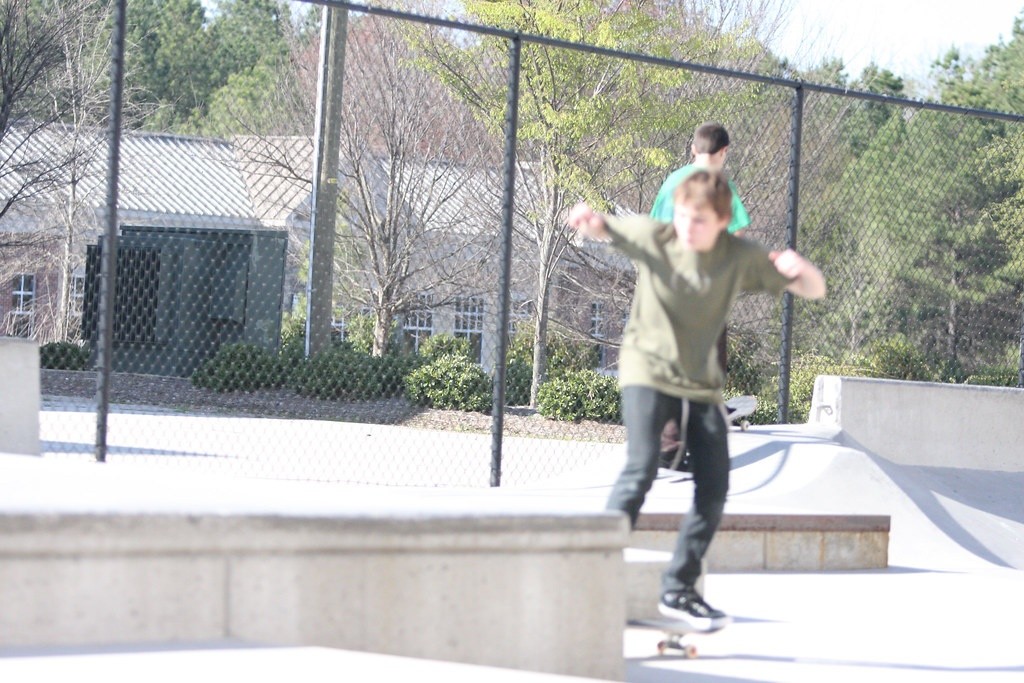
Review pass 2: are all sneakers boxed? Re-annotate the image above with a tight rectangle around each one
[656,590,728,632]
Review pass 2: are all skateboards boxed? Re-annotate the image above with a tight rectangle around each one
[660,392,758,460]
[623,613,723,659]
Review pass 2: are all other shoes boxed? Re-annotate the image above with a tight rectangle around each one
[656,447,691,472]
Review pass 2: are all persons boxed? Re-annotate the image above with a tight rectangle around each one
[567,172,827,630]
[647,120,748,236]
